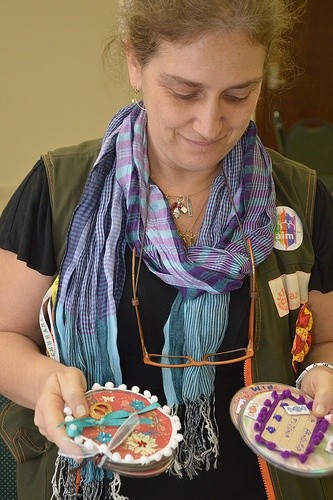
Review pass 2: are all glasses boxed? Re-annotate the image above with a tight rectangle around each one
[132,238,256,368]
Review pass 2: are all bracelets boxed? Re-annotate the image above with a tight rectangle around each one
[295,362,333,389]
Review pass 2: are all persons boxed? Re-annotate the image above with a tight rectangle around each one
[1,0,333,500]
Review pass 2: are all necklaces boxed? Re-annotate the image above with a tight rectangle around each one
[164,184,211,247]
